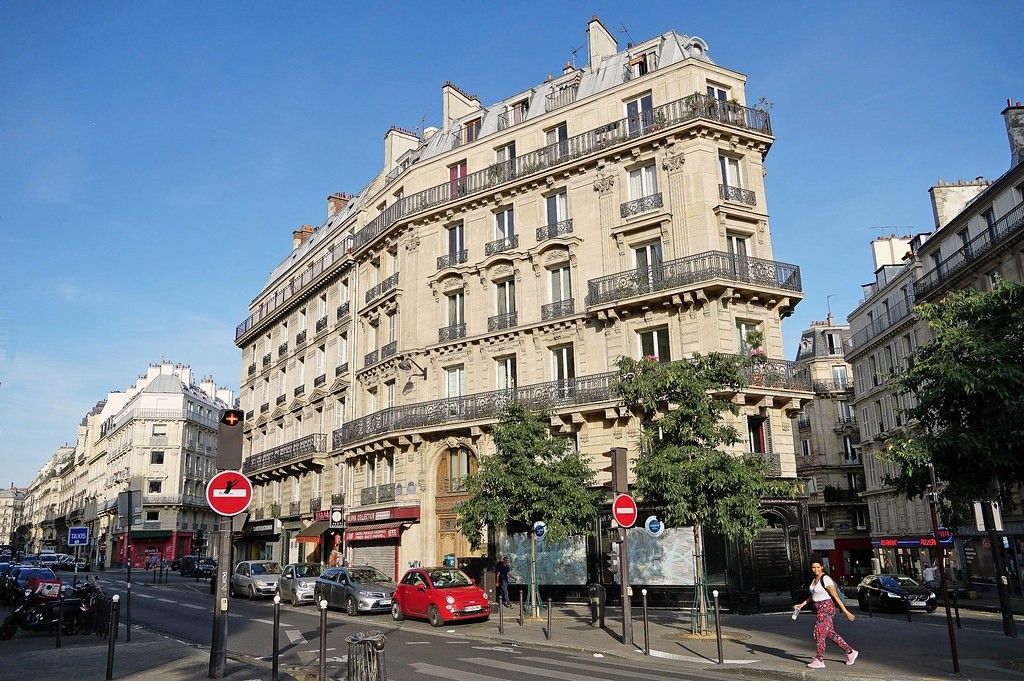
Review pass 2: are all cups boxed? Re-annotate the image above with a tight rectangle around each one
[792,608,800,619]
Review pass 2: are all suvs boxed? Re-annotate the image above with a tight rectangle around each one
[144,553,166,567]
[180,556,216,578]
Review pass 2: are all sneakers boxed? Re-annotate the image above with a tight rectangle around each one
[806,656,826,669]
[844,649,858,665]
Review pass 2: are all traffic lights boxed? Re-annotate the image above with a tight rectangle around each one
[216,409,244,470]
[206,471,253,517]
[602,447,628,494]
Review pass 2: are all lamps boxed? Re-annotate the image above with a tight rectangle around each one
[398,357,427,381]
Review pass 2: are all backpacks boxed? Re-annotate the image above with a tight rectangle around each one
[495,561,504,578]
[820,574,846,613]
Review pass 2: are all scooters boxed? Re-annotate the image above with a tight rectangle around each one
[0,582,89,641]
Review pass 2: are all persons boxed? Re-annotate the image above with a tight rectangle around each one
[496,557,519,610]
[793,558,858,669]
[923,565,938,593]
[144,554,167,572]
[82,553,107,569]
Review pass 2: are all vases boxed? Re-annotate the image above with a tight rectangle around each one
[752,355,767,362]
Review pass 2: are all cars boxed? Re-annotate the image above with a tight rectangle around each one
[391,566,491,627]
[313,566,397,616]
[233,560,284,601]
[857,573,938,615]
[171,557,183,571]
[274,562,326,607]
[0,543,91,607]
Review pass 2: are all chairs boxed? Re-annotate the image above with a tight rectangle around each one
[306,569,317,575]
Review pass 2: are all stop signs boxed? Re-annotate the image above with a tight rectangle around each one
[100,544,107,549]
[612,494,638,529]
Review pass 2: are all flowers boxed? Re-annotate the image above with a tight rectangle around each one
[742,329,766,355]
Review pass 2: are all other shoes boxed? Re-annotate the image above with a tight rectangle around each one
[508,604,513,609]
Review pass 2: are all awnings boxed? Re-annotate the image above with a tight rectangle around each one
[345,522,402,541]
[295,520,330,544]
